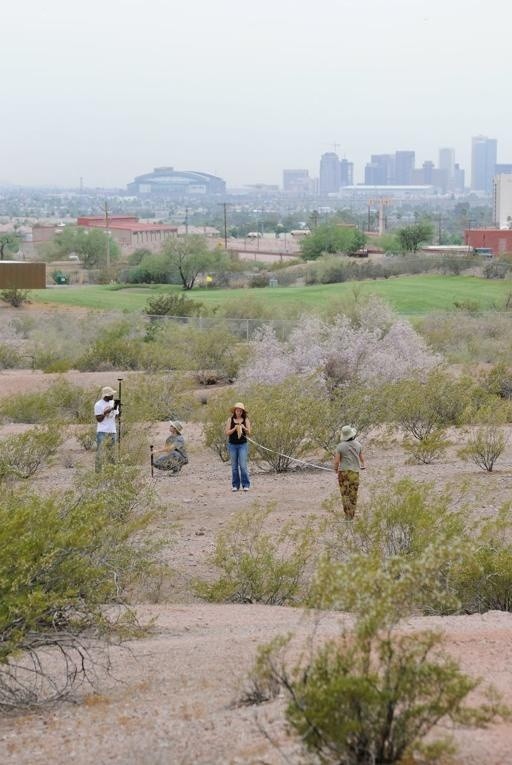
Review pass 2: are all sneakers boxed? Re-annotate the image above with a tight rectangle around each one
[232,487,238,492]
[169,470,183,477]
[243,487,250,491]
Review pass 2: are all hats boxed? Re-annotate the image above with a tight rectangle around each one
[169,419,183,436]
[339,425,357,442]
[100,387,117,400]
[230,401,248,413]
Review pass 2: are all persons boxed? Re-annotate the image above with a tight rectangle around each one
[151,421,188,476]
[93,385,122,473]
[225,401,251,491]
[334,424,366,521]
